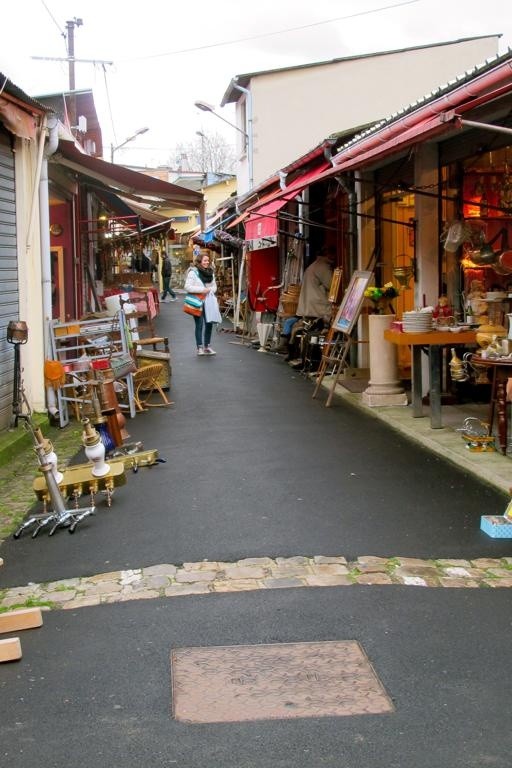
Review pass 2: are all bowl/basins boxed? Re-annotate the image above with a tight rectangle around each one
[436,326,470,333]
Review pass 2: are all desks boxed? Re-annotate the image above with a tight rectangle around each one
[472,357,512,457]
[383,329,478,429]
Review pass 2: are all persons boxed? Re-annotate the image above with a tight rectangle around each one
[158,251,179,303]
[278,243,337,373]
[131,248,159,286]
[184,252,219,355]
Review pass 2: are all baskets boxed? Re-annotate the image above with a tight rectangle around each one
[280,283,302,314]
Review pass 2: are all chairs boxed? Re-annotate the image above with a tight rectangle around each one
[117,292,174,413]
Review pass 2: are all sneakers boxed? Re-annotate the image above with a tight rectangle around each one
[196,347,216,355]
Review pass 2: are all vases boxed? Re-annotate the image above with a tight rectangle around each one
[367,315,404,395]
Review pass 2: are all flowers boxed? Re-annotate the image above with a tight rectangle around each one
[363,281,400,314]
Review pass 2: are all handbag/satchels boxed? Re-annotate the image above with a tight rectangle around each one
[182,292,206,317]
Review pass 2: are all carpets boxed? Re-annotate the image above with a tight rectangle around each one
[336,378,370,393]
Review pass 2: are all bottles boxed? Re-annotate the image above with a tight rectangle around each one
[506,377,512,402]
[479,192,487,218]
[450,348,464,380]
[466,299,474,325]
[486,335,502,359]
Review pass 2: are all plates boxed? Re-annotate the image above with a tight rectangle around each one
[476,299,503,302]
[402,312,433,334]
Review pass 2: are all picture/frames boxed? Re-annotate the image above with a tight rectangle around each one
[50,246,66,325]
[330,268,373,335]
